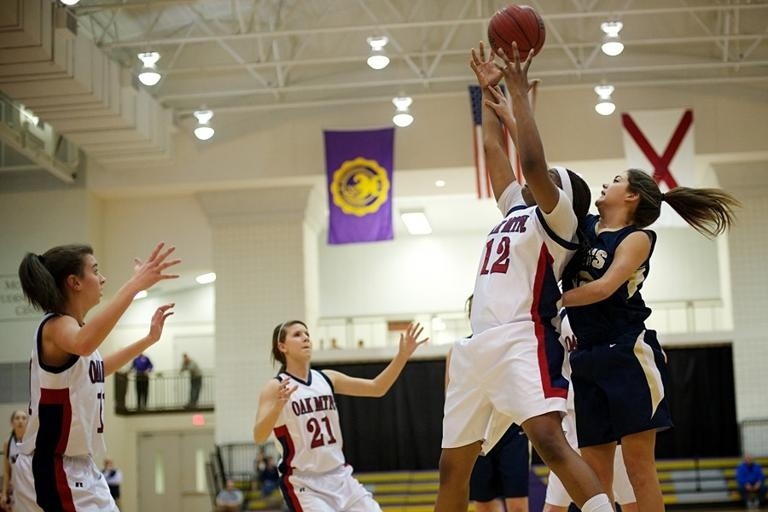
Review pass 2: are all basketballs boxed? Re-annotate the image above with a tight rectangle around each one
[488,5,546,63]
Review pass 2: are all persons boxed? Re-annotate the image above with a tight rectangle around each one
[11,240,182,511]
[253,319,430,512]
[3,408,28,505]
[178,353,203,409]
[735,454,768,508]
[126,352,153,411]
[255,447,280,496]
[100,457,123,499]
[216,478,244,512]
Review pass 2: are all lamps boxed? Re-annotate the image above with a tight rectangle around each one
[390,93,414,128]
[191,104,216,141]
[599,17,624,59]
[137,42,161,88]
[365,28,390,70]
[595,77,616,117]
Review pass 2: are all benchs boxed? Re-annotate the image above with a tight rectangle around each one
[353,470,440,512]
[654,457,767,509]
